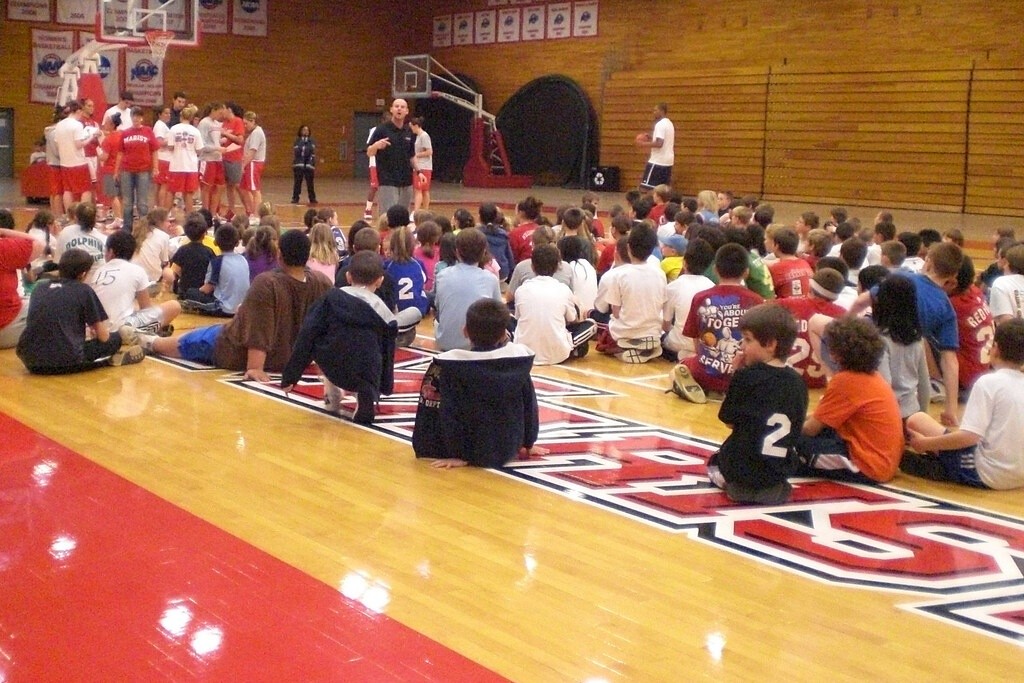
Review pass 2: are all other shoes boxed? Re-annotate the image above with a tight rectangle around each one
[212,214,228,226]
[622,346,663,363]
[95,215,106,221]
[291,200,298,203]
[157,324,174,337]
[899,450,944,480]
[193,199,202,205]
[351,392,374,423]
[728,481,791,506]
[105,218,123,229]
[363,210,372,219]
[225,210,235,223]
[618,336,661,349]
[323,381,342,410]
[310,199,318,203]
[665,364,706,404]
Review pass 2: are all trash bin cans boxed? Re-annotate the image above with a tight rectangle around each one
[589,165,621,192]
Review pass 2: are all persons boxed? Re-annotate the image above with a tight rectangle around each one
[91,230,181,335]
[433,227,502,352]
[905,318,1023,490]
[181,222,251,317]
[291,125,318,203]
[411,298,550,469]
[707,304,810,503]
[280,250,398,426]
[377,196,671,362]
[794,316,904,483]
[0,203,398,382]
[671,242,766,403]
[634,101,676,194]
[625,185,1024,443]
[514,241,597,364]
[366,99,427,218]
[408,115,433,222]
[30,92,266,231]
[16,249,146,377]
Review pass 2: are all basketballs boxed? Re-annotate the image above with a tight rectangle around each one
[637,134,648,145]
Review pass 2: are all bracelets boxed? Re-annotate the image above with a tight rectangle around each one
[417,169,423,174]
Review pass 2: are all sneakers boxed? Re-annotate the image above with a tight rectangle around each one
[108,345,145,366]
[119,325,160,354]
